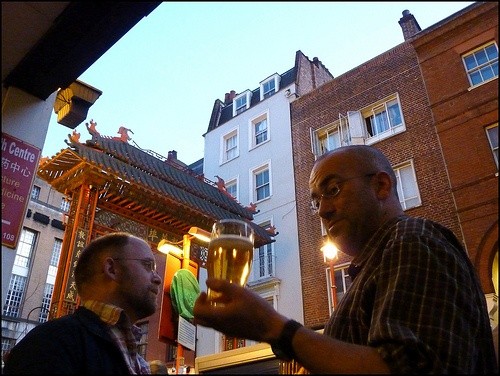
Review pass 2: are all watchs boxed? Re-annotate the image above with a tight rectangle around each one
[271,319,302,362]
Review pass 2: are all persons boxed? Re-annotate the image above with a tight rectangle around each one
[2,230,161,376]
[191,146,497,375]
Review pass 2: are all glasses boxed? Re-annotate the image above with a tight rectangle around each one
[311,171,393,214]
[107,256,158,274]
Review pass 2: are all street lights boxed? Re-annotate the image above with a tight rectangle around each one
[157,227,211,376]
[322,242,337,311]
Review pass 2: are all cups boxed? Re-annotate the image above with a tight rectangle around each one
[207,218,255,308]
